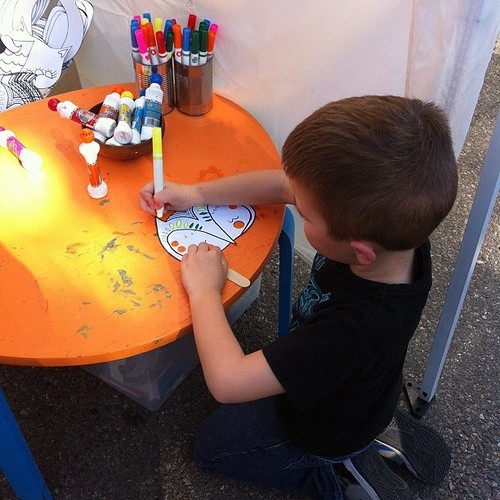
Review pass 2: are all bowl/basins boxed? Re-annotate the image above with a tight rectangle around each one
[81,99,166,161]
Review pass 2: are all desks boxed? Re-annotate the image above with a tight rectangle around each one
[0,81,296,500]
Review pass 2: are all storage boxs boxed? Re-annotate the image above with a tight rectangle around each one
[79,271,263,411]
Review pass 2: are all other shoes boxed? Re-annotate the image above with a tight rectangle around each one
[371,407,452,486]
[334,443,411,500]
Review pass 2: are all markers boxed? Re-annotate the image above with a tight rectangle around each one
[130,13,219,65]
[152,127,165,219]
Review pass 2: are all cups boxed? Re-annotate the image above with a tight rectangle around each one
[174,56,213,116]
[131,54,174,115]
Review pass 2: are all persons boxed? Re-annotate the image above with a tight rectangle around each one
[139,95,459,500]
